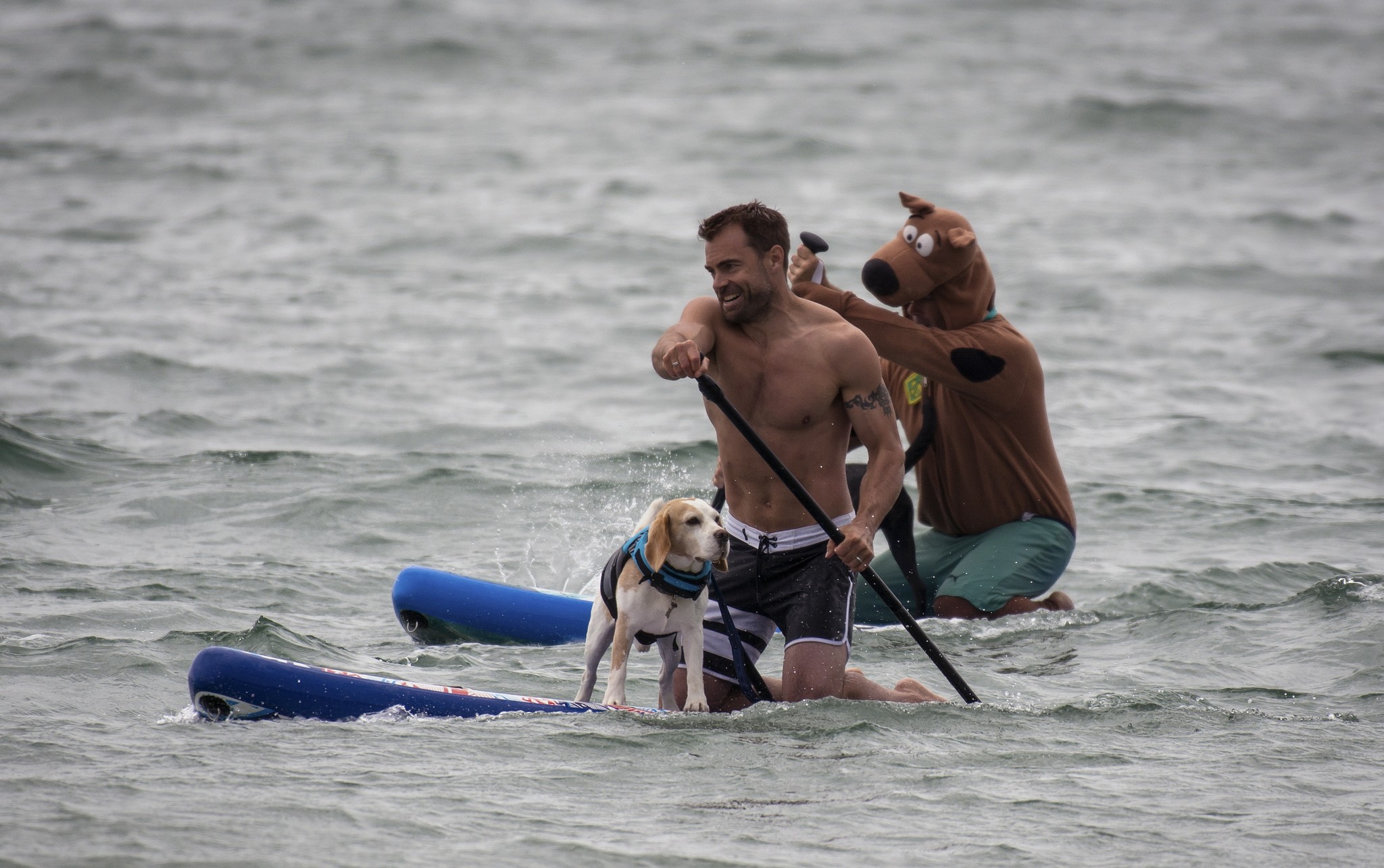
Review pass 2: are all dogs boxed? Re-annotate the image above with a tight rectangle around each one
[571,491,732,714]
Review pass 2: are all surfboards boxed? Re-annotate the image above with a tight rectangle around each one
[390,567,593,643]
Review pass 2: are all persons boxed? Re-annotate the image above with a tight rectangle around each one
[787,191,1077,620]
[652,198,947,712]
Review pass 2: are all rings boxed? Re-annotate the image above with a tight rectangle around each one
[672,362,679,366]
[856,557,862,563]
[864,564,866,566]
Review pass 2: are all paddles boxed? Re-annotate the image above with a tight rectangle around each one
[693,370,981,705]
[712,231,830,513]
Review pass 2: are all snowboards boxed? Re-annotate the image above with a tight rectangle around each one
[190,647,722,725]
